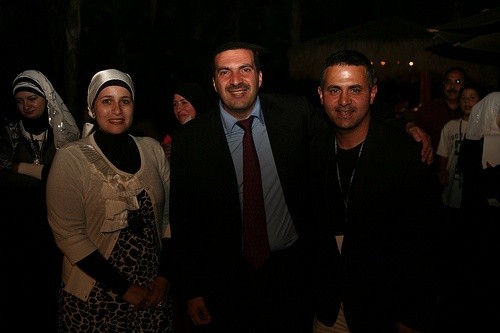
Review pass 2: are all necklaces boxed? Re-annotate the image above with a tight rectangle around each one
[26,115,47,164]
[334,137,367,208]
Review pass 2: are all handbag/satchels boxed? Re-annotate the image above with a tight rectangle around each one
[441,169,463,208]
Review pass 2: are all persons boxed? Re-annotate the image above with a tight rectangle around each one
[162,82,212,160]
[170,39,433,333]
[419,67,471,139]
[306,50,443,333]
[0,70,80,231]
[46,69,177,333]
[436,85,482,195]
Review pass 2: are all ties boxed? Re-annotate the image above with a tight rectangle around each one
[235,115,272,268]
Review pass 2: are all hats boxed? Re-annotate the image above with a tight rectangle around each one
[87,68,135,116]
